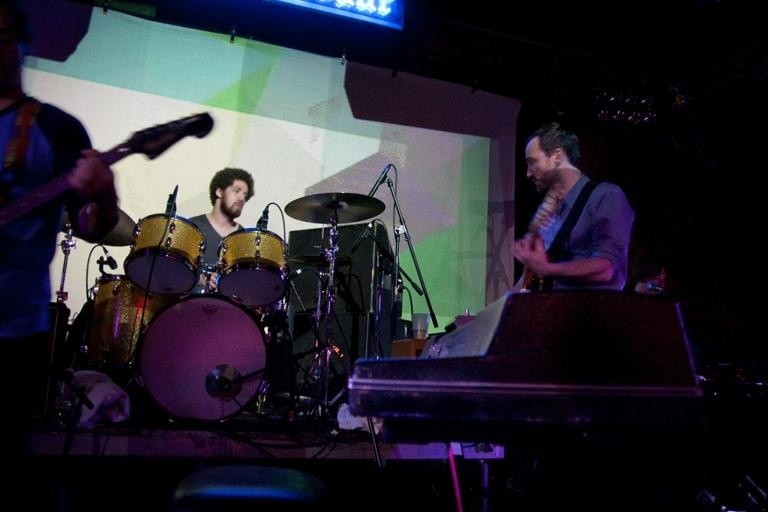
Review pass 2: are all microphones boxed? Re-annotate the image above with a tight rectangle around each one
[367,165,390,197]
[255,209,268,230]
[166,194,177,213]
[104,247,119,269]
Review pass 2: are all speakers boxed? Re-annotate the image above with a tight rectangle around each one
[289,227,401,317]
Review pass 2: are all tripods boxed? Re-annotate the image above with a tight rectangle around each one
[296,228,346,418]
[328,232,385,471]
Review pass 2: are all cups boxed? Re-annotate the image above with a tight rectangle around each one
[411,313,428,340]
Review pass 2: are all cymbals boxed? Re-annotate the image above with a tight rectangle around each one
[284,192,385,223]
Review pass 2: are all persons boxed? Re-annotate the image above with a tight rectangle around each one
[188,167,254,294]
[0,1,119,512]
[509,121,636,291]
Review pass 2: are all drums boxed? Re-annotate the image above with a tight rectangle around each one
[134,288,270,428]
[123,213,205,296]
[82,274,177,372]
[215,228,289,308]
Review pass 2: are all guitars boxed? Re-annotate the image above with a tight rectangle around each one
[521,183,564,291]
[0,112,213,226]
